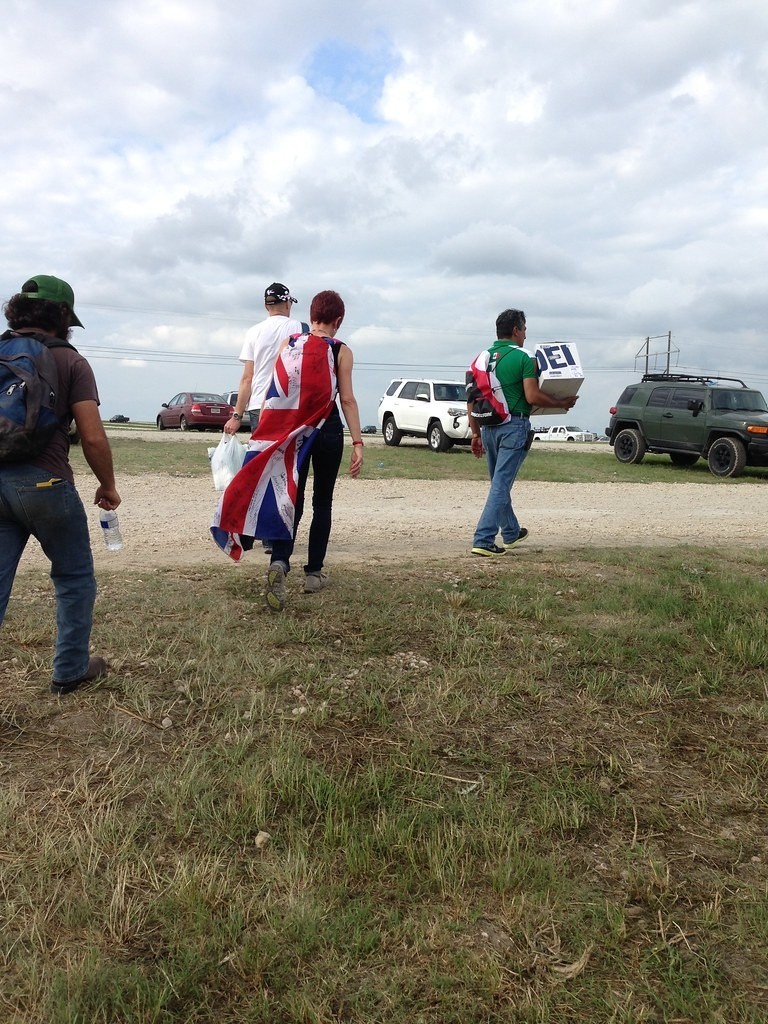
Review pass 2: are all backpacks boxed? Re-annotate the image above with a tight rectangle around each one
[0,329,78,464]
[471,346,524,427]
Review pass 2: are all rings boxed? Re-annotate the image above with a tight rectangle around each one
[358,463,362,467]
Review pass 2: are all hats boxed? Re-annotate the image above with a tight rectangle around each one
[21,275,85,329]
[264,283,298,305]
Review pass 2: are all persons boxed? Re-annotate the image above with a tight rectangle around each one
[0,274,121,694]
[259,290,364,611]
[466,307,579,557]
[224,283,309,553]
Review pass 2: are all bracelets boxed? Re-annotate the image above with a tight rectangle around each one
[352,441,363,446]
[472,433,481,438]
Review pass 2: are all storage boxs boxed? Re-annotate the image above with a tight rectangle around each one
[531,341,586,416]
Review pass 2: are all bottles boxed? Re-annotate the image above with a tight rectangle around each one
[99,508,123,551]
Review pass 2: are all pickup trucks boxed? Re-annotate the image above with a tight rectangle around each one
[110,415,129,423]
[533,426,593,442]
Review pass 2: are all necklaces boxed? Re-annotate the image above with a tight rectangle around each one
[312,328,329,334]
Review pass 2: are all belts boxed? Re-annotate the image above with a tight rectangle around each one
[511,412,529,418]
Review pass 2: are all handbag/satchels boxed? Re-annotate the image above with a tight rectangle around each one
[208,432,245,490]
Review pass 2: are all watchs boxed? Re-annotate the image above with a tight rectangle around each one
[232,412,243,420]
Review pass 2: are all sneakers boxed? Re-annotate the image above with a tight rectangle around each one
[50,657,106,694]
[471,544,507,557]
[265,561,288,611]
[503,528,528,549]
[304,573,329,592]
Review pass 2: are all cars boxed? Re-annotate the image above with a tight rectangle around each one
[157,392,234,431]
[361,426,376,434]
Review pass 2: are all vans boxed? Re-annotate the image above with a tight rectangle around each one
[221,391,250,430]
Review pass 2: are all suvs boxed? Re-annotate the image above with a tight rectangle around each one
[605,373,768,478]
[377,377,481,453]
